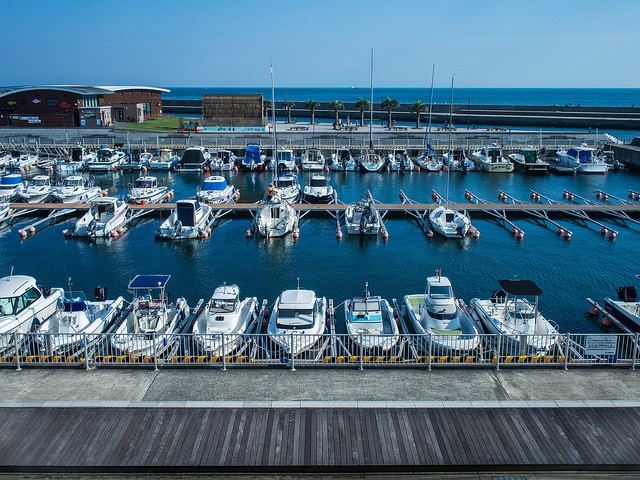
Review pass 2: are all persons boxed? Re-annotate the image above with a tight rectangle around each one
[267,183,275,202]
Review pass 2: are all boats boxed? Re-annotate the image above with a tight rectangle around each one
[257,192,296,236]
[268,149,296,171]
[120,153,152,170]
[273,176,300,203]
[196,175,235,203]
[36,159,55,168]
[125,175,168,203]
[149,149,179,170]
[303,175,333,203]
[556,143,608,172]
[301,148,325,170]
[469,279,559,354]
[72,196,128,236]
[154,199,214,239]
[0,265,64,352]
[412,145,443,171]
[471,146,514,172]
[0,150,12,167]
[192,281,259,356]
[428,202,471,238]
[507,147,549,176]
[51,175,101,202]
[267,276,326,356]
[0,173,23,201]
[173,146,211,172]
[241,142,265,170]
[34,276,123,354]
[603,286,639,325]
[404,268,480,355]
[210,149,238,170]
[442,149,474,170]
[343,281,399,352]
[109,274,189,356]
[328,147,357,170]
[357,152,385,170]
[344,200,380,234]
[385,149,413,170]
[87,147,125,169]
[20,174,51,203]
[5,153,38,169]
[540,146,582,172]
[604,150,625,169]
[55,149,93,171]
